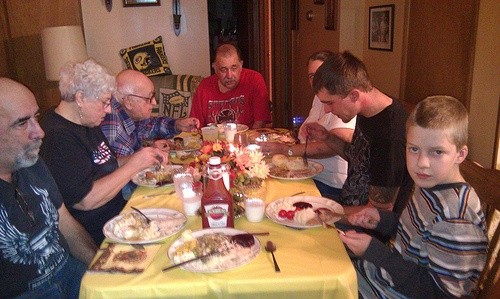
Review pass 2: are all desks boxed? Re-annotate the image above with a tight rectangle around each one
[79,128,358,299]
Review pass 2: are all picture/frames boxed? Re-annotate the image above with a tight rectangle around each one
[368,4,396,51]
[325,0,337,30]
[123,0,160,7]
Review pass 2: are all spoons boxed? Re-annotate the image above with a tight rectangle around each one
[265,240,281,272]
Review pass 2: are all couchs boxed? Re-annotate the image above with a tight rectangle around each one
[147,74,206,118]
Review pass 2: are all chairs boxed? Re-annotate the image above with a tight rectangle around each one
[460,160,500,299]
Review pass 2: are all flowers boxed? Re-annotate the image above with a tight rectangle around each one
[187,141,270,186]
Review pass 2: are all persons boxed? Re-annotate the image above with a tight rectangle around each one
[372,14,388,42]
[99,68,200,203]
[190,45,271,132]
[0,76,99,299]
[300,52,415,213]
[38,58,169,250]
[338,96,489,299]
[258,49,356,200]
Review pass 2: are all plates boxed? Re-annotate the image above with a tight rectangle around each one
[102,208,189,245]
[266,195,345,228]
[168,136,203,152]
[265,158,325,181]
[131,164,182,188]
[168,226,262,273]
[216,123,249,134]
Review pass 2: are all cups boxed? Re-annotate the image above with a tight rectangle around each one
[201,126,219,141]
[234,132,251,147]
[172,165,202,218]
[241,186,267,222]
[223,121,237,142]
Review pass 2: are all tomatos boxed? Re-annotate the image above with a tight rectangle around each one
[279,208,301,219]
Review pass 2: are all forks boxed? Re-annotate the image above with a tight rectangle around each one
[302,135,308,166]
[314,212,346,235]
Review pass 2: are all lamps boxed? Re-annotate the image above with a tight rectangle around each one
[40,26,90,81]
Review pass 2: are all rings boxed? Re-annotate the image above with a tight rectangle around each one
[267,152,269,156]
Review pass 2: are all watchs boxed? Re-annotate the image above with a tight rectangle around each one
[288,145,293,156]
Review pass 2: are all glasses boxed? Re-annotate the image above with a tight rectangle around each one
[92,94,113,109]
[128,92,156,104]
[307,74,315,80]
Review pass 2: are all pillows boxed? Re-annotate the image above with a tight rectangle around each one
[158,87,193,120]
[120,35,172,78]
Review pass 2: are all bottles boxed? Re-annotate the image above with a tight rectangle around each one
[200,157,234,228]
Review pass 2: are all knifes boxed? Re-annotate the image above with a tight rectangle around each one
[318,209,378,225]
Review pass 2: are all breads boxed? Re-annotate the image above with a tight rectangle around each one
[272,154,310,171]
